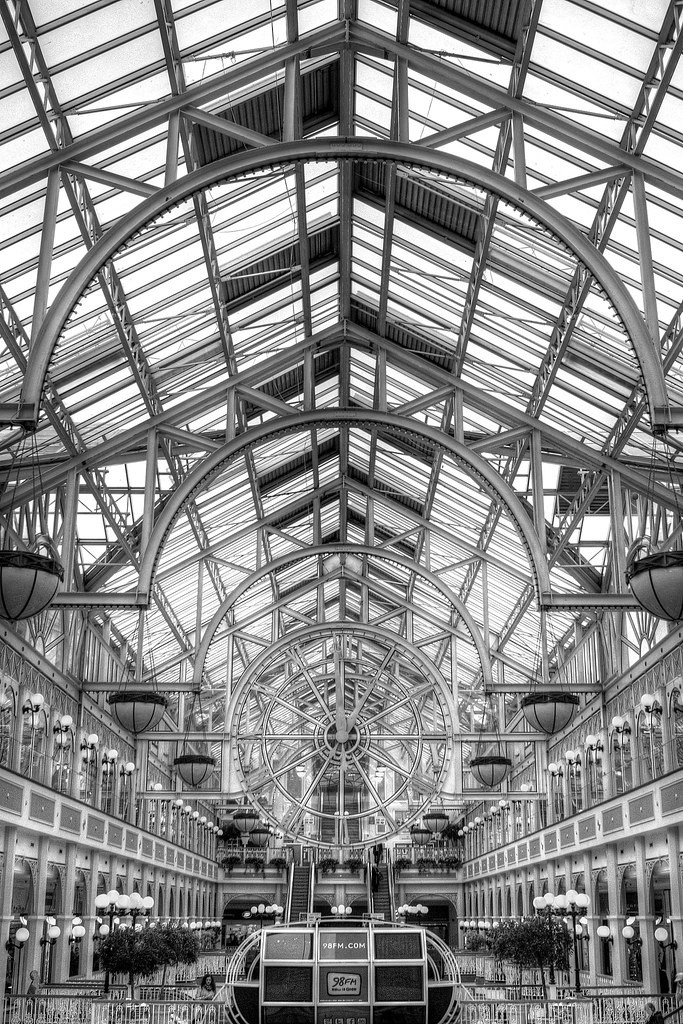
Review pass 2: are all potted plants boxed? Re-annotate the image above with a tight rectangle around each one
[98,919,199,999]
[485,917,573,1024]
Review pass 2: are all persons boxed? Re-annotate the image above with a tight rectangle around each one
[26,970,42,1024]
[644,1003,665,1024]
[194,974,218,1024]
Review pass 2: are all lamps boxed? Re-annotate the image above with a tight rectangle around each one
[625,426,683,620]
[0,427,64,624]
[109,606,166,735]
[0,690,683,953]
[520,604,579,733]
